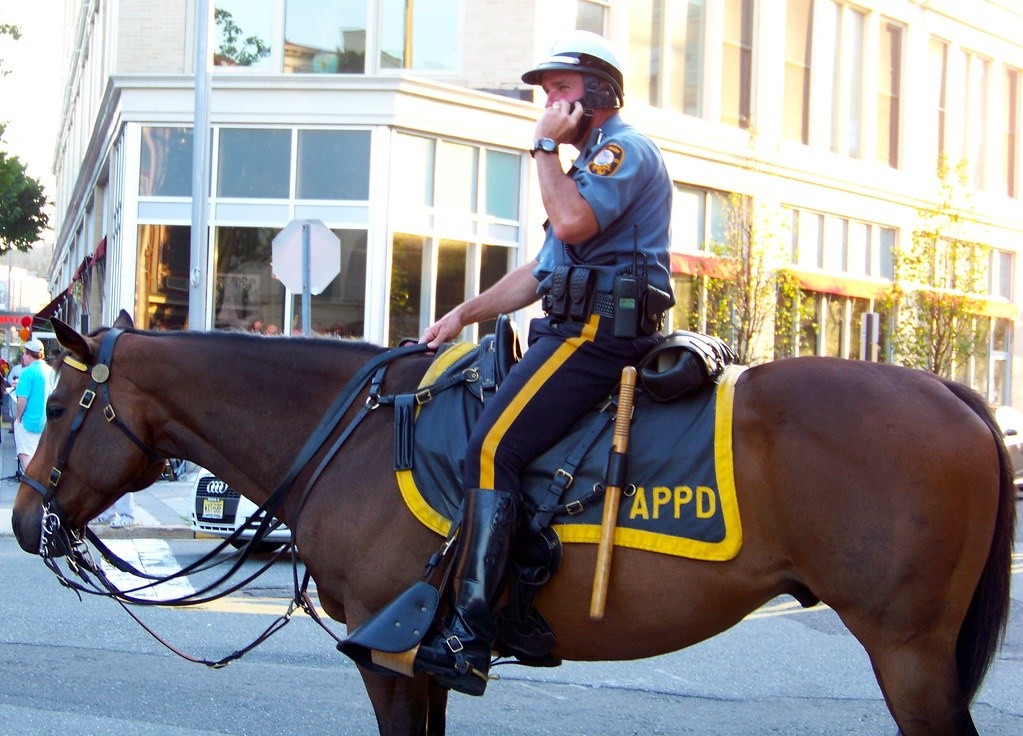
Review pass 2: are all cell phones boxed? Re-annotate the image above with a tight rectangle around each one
[569,97,588,116]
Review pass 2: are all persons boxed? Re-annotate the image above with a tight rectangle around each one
[357,31,674,695]
[248,313,300,335]
[0,340,61,473]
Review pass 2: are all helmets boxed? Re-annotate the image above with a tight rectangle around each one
[523,30,626,109]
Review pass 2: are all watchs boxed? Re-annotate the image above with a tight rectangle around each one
[529,138,559,158]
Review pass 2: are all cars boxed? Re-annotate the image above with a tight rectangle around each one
[189,466,295,557]
[993,404,1023,494]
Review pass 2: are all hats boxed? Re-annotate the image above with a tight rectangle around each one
[25,340,45,355]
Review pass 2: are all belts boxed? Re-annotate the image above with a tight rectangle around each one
[542,292,665,331]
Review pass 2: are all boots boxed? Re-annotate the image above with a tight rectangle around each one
[365,487,518,698]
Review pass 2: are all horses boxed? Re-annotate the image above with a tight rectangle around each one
[10,309,1016,736]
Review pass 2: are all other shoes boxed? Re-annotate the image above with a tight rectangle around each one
[8,429,14,433]
